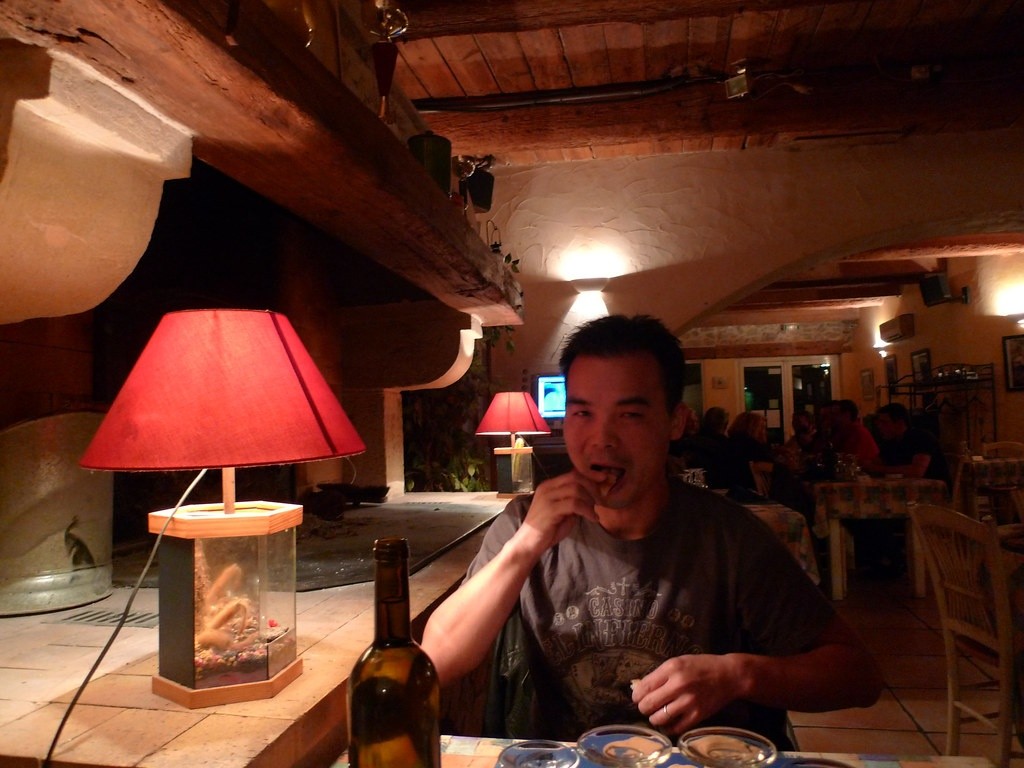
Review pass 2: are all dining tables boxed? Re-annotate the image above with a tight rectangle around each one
[324,733,994,768]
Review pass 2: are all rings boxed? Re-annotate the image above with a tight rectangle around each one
[664,705,671,720]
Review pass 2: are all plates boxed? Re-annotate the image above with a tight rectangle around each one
[577,725,672,768]
[678,727,777,768]
[498,740,580,768]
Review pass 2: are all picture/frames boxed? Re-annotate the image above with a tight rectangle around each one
[883,354,899,393]
[859,367,874,401]
[1002,334,1024,392]
[910,348,936,393]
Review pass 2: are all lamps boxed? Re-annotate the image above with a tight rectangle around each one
[571,276,609,292]
[80,308,366,712]
[475,391,551,447]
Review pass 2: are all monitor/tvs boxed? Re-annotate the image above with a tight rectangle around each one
[531,373,567,430]
[916,272,952,307]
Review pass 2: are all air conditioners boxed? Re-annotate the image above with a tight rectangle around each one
[879,313,915,345]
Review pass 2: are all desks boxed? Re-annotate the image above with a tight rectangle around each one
[797,473,947,599]
[740,500,816,577]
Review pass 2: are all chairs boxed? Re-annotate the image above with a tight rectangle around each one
[906,442,1024,768]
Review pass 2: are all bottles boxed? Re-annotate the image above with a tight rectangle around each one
[344,537,440,768]
[822,442,838,470]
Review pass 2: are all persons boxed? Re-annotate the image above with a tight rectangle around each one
[420,314,882,752]
[796,399,951,480]
[670,406,775,490]
